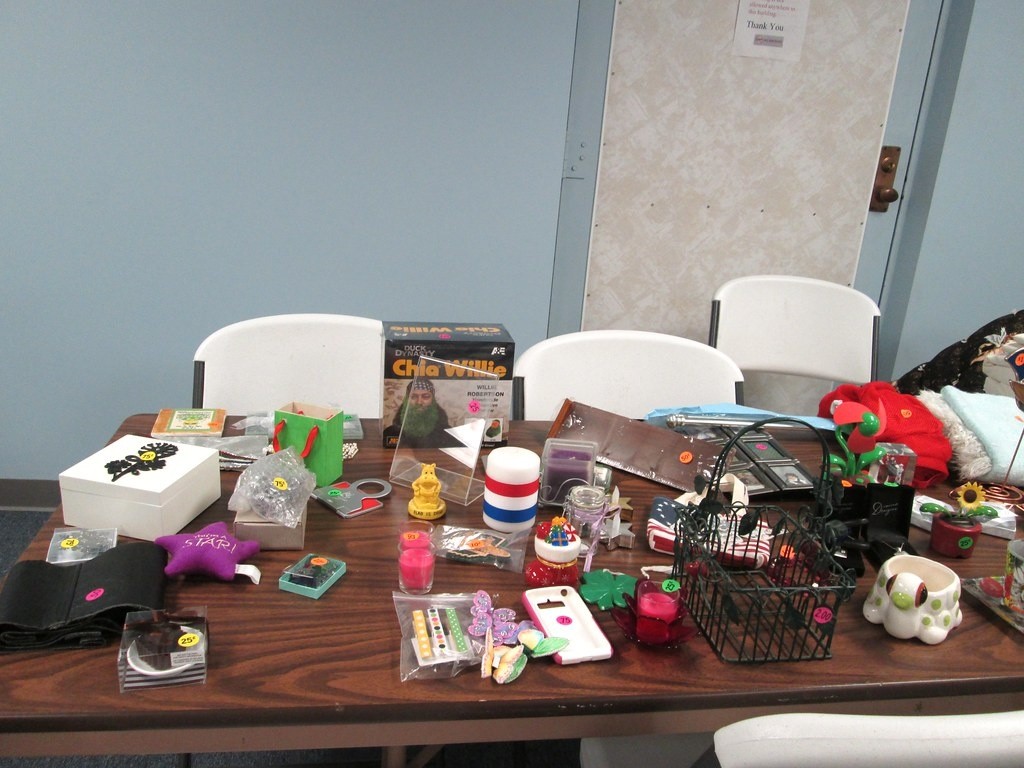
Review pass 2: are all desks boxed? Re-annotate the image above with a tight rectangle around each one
[1,392,1023,768]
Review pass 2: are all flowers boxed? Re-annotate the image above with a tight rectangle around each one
[918,481,999,526]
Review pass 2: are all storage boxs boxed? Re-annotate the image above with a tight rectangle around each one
[278,553,346,599]
[383,317,516,448]
[116,605,208,693]
[55,436,222,541]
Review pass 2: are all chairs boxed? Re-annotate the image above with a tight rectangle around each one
[578,700,1024,768]
[194,309,383,428]
[708,273,882,409]
[515,326,743,425]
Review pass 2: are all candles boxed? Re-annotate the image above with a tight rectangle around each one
[539,437,599,506]
[636,580,680,647]
